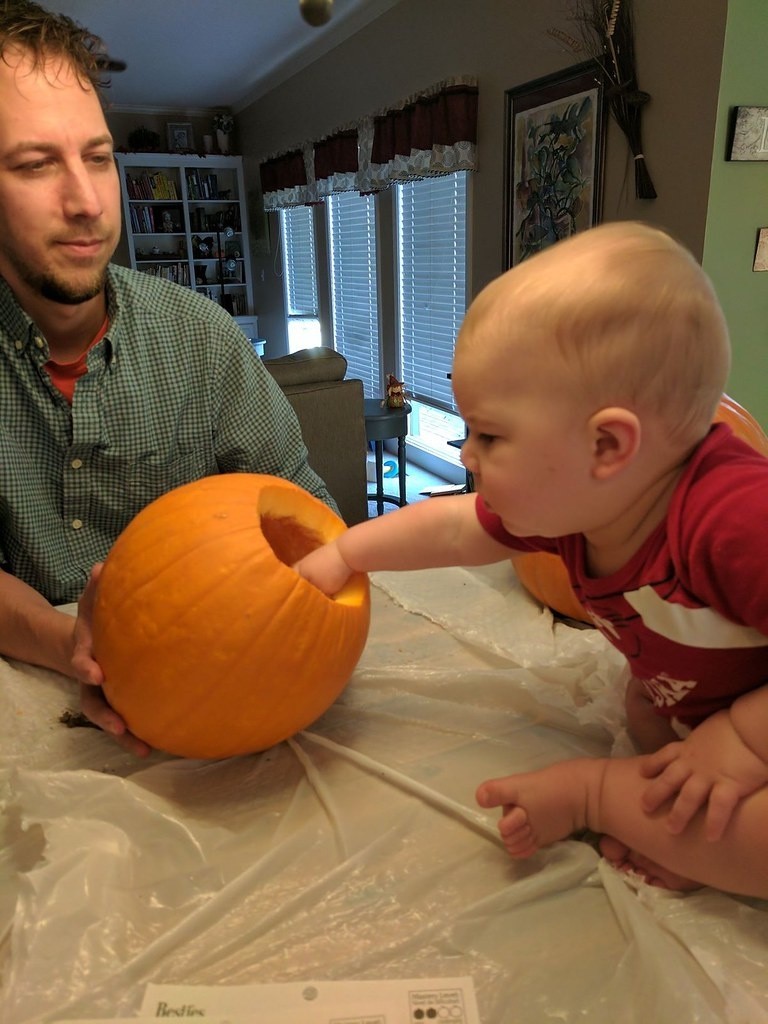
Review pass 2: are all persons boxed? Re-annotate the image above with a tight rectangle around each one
[381,375,405,408]
[292,221,767,899]
[0,0,345,759]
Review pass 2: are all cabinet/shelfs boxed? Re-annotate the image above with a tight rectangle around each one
[111,150,259,341]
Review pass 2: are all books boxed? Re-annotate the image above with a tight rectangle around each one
[129,203,155,233]
[140,262,190,286]
[197,288,248,316]
[191,205,241,232]
[125,170,178,200]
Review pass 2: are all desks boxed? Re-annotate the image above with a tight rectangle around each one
[365,396,412,521]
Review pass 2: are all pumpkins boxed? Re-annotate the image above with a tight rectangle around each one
[510,393,768,624]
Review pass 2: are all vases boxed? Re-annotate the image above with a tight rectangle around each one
[216,128,229,154]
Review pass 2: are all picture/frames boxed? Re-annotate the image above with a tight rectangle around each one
[500,52,611,276]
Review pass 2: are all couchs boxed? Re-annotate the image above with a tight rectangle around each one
[259,345,368,530]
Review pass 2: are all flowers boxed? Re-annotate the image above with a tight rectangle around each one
[211,112,237,133]
